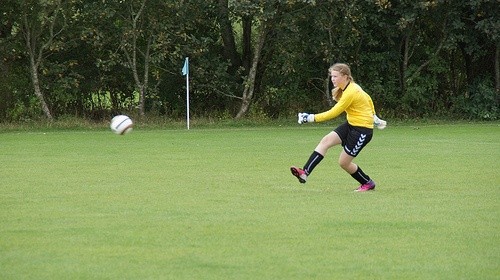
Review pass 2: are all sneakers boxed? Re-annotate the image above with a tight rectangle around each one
[353,180,375,192]
[289,165,307,183]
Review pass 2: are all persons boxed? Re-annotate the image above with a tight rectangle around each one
[289,62,388,193]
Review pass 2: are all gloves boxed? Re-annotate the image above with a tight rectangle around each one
[373,115,387,129]
[297,112,315,124]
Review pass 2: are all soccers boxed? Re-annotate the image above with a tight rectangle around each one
[111,115,133,135]
